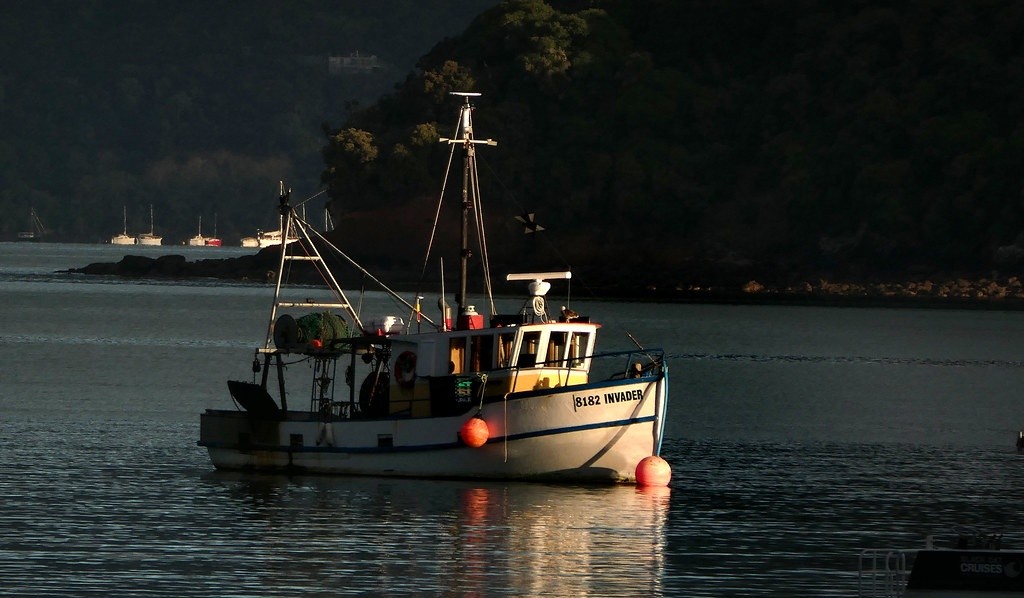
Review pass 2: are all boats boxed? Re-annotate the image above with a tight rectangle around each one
[197,91,668,486]
[189,215,223,247]
[240,230,302,248]
[111,204,162,246]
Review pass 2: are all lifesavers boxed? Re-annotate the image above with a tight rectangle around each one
[394,350,417,389]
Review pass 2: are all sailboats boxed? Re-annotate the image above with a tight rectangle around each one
[17,206,47,239]
